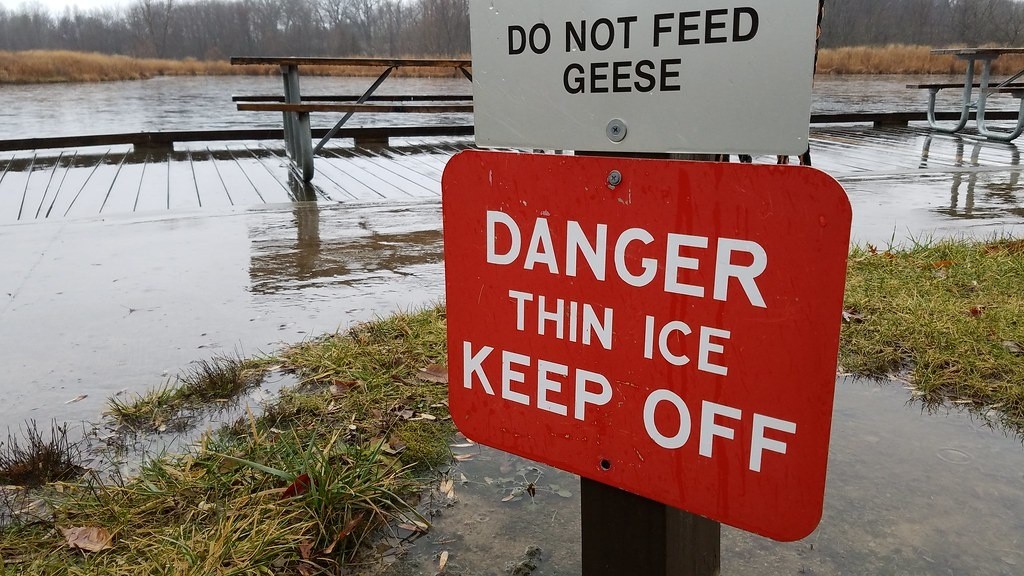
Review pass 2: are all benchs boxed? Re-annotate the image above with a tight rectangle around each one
[231,93,474,160]
[982,87,1024,141]
[905,81,1024,132]
[236,101,475,182]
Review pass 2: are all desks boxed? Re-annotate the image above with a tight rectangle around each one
[229,56,472,169]
[929,47,1024,131]
